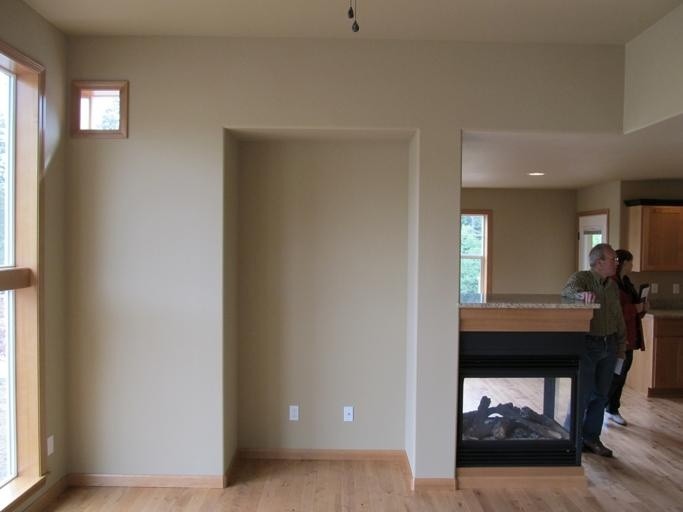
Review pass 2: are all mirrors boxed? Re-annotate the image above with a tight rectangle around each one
[69,78,129,140]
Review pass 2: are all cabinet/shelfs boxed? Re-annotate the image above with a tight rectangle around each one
[624,205,683,272]
[624,308,683,398]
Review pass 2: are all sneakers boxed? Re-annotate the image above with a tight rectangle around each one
[604,410,628,425]
[580,438,613,457]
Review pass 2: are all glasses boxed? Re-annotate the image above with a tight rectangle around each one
[603,256,619,263]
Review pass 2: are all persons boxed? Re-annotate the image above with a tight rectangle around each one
[561,241,628,458]
[605,247,650,427]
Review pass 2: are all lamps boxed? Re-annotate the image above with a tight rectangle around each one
[351,0,359,33]
[347,0,354,19]
